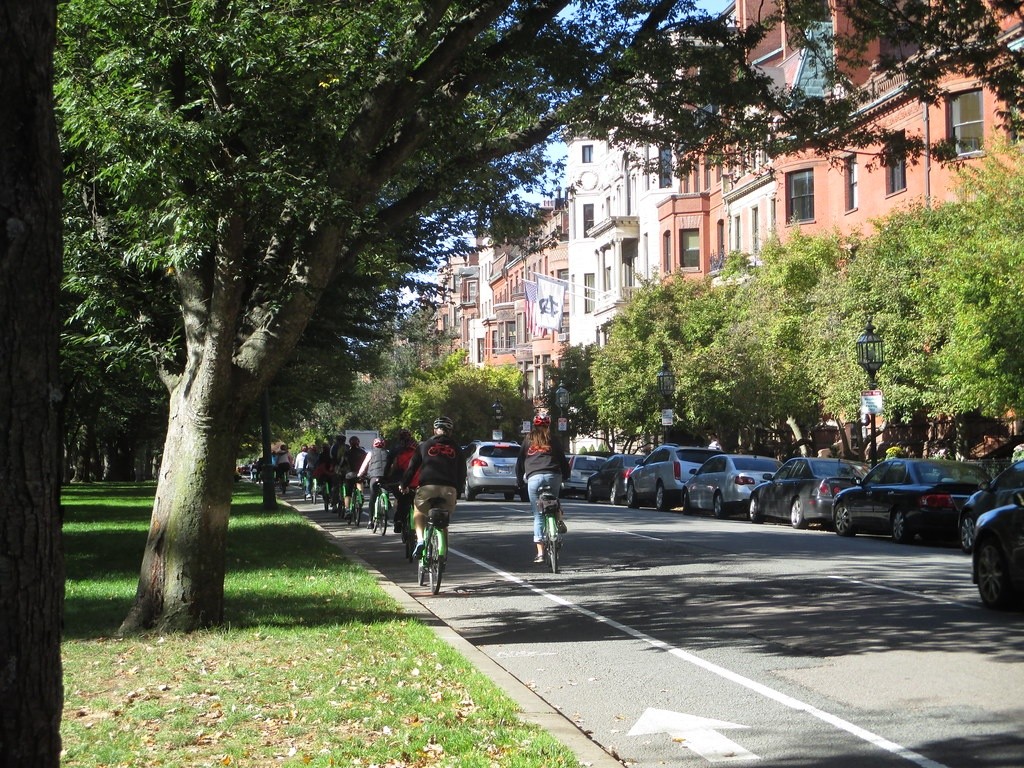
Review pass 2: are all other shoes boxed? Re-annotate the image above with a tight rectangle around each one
[344,510,350,520]
[412,540,424,556]
[306,493,311,498]
[534,554,545,563]
[395,520,402,533]
[367,523,373,529]
[556,520,567,533]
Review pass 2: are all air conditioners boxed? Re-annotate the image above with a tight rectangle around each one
[558,333,569,342]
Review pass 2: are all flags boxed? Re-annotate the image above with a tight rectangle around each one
[534,276,566,332]
[524,280,549,338]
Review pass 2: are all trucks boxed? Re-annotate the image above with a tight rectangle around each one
[345,429,378,451]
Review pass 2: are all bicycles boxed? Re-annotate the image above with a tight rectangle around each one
[250,468,389,538]
[533,484,564,573]
[402,486,447,596]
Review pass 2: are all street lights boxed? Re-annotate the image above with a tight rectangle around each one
[555,379,571,455]
[856,317,884,466]
[656,364,675,442]
[492,398,503,429]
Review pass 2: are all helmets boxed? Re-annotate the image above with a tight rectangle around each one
[310,445,317,452]
[373,438,385,448]
[533,412,551,426]
[433,416,454,430]
[399,429,411,441]
[302,445,308,451]
[349,436,360,446]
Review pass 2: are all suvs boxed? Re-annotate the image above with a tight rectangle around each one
[626,443,727,512]
[457,440,530,503]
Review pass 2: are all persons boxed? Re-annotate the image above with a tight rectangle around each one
[382,428,422,533]
[515,412,570,564]
[248,434,390,529]
[398,416,468,571]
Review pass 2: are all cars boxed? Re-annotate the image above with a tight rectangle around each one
[586,455,648,505]
[830,456,993,545]
[748,456,880,529]
[681,453,784,518]
[559,454,608,497]
[958,458,1024,615]
